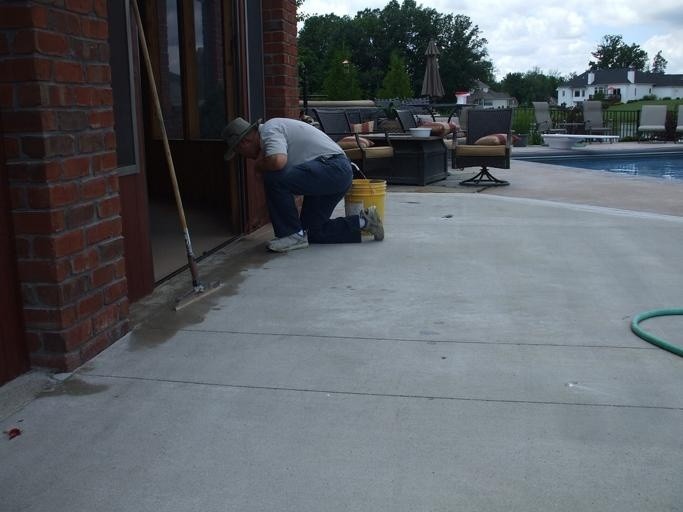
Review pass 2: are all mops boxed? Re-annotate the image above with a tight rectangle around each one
[133,1,223,313]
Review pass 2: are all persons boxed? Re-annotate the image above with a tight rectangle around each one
[217,115,385,253]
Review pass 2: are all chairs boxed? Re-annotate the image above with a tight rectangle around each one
[528,100,683,144]
[310,105,514,185]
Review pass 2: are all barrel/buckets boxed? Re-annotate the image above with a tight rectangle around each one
[344,162,386,234]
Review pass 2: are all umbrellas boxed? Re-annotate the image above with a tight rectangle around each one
[418,38,445,107]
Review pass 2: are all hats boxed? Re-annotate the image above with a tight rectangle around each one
[219,117,262,161]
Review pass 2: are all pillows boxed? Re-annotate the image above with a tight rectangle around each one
[473,134,521,145]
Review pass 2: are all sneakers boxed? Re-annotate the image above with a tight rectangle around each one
[266,231,309,252]
[360,205,384,242]
